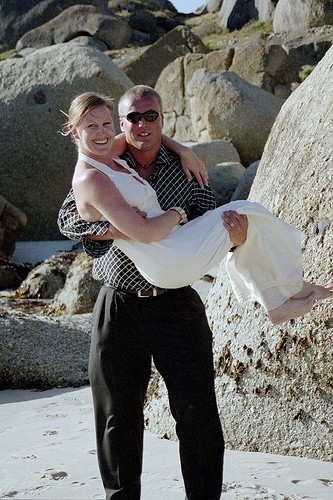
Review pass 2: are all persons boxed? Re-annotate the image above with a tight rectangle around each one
[0,195,27,268]
[56,85,249,500]
[67,92,332,327]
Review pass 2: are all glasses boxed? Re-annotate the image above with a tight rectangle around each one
[122,110,163,123]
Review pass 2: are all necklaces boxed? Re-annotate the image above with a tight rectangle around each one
[128,152,157,170]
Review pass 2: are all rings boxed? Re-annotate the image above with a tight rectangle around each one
[229,223,234,227]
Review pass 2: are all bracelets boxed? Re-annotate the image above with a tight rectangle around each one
[166,207,186,223]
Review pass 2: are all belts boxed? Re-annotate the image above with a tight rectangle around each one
[126,289,168,298]
